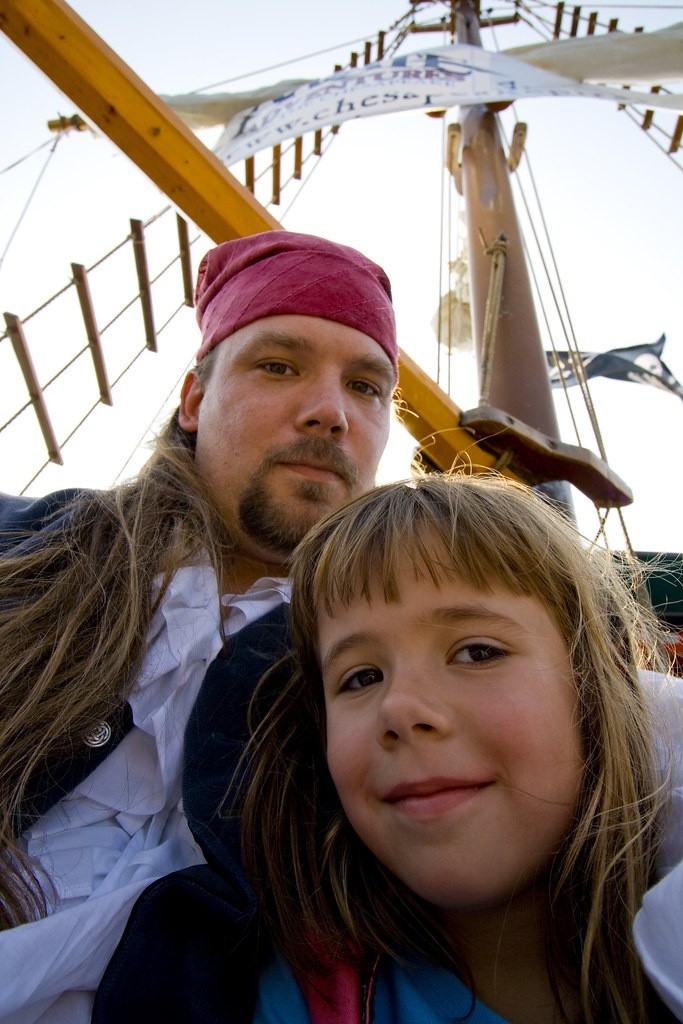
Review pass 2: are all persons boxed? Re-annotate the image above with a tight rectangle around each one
[248,459,683,1024]
[0,231,683,1024]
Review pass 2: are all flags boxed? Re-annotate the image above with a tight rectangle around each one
[547,332,683,398]
